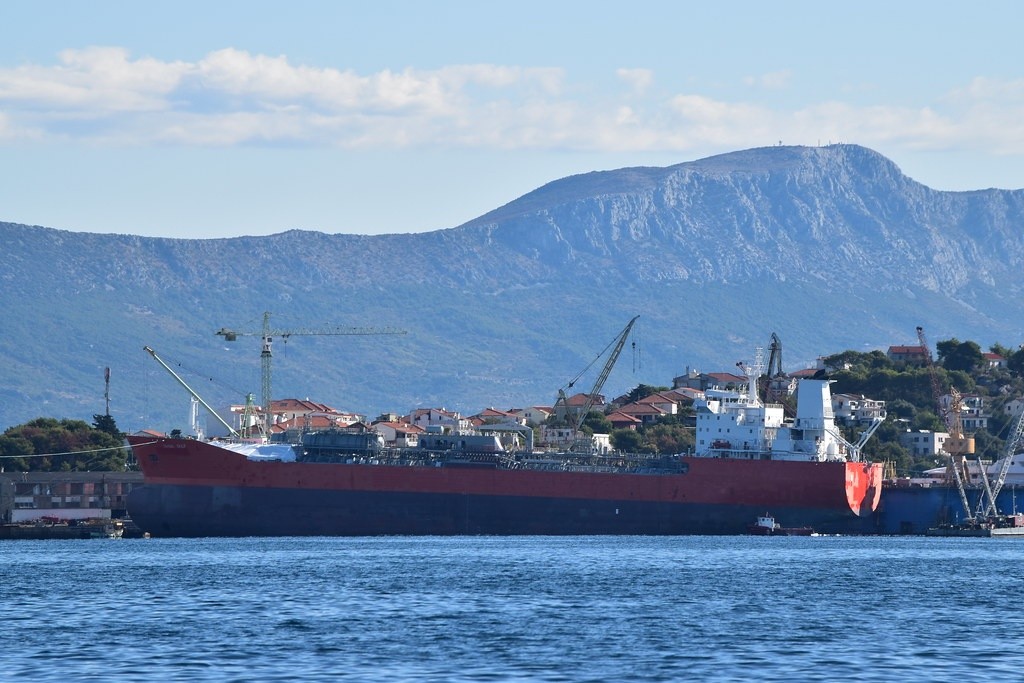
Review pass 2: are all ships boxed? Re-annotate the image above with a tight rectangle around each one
[120,350,887,536]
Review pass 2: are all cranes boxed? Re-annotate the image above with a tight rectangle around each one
[553,314,640,432]
[219,309,412,443]
[915,327,960,435]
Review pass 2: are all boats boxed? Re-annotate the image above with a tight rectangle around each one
[930,454,1023,538]
[747,512,817,537]
[0,515,124,540]
